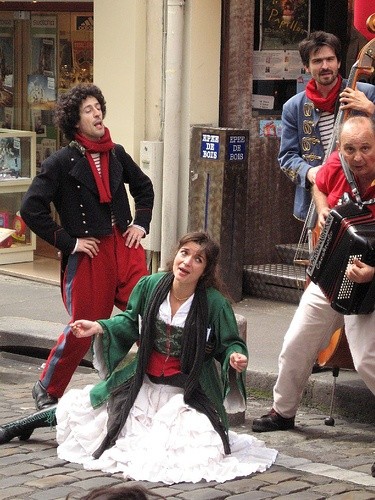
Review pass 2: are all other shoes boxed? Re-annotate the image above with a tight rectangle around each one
[312,361,332,374]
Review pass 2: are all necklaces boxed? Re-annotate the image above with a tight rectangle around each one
[171,290,188,300]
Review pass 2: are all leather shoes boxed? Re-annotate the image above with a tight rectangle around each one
[32,380,58,412]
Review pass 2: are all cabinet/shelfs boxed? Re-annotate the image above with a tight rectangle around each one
[0,128,37,266]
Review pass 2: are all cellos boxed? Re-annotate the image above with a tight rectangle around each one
[292,13,375,426]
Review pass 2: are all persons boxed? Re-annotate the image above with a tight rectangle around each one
[252,116,375,477]
[0,230,278,484]
[278,31,375,222]
[18,84,155,411]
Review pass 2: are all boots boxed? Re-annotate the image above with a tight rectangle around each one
[0,403,58,445]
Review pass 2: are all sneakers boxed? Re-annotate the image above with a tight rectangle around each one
[371,462,375,478]
[253,408,295,431]
